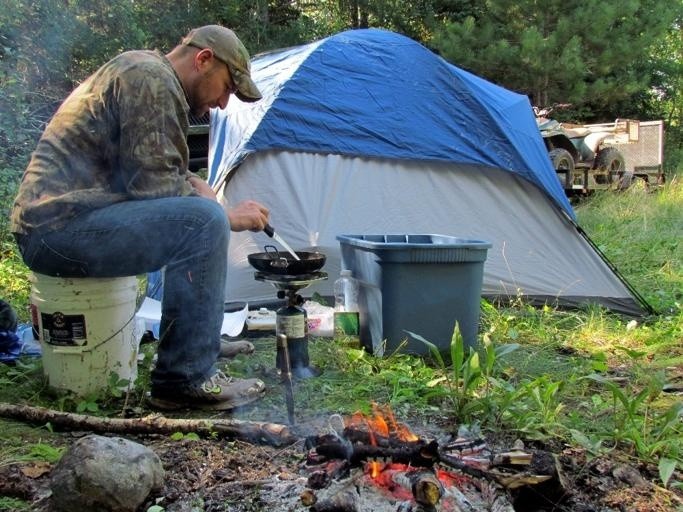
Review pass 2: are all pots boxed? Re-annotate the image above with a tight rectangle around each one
[247,245,327,271]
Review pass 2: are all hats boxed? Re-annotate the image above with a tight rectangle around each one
[183,24,263,104]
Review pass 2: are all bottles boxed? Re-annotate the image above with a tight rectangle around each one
[333,270,362,350]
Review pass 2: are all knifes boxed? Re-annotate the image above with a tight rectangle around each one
[275,334,300,424]
[260,222,308,261]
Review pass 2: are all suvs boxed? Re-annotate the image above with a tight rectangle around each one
[531,103,627,191]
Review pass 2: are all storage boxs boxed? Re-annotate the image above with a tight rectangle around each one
[341,235,491,361]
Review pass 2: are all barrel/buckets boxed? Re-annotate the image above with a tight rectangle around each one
[34,271,138,400]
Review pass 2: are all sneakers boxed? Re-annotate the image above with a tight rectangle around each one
[220,338,255,356]
[151,370,267,411]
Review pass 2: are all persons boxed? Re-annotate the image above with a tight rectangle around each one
[8,26,273,408]
